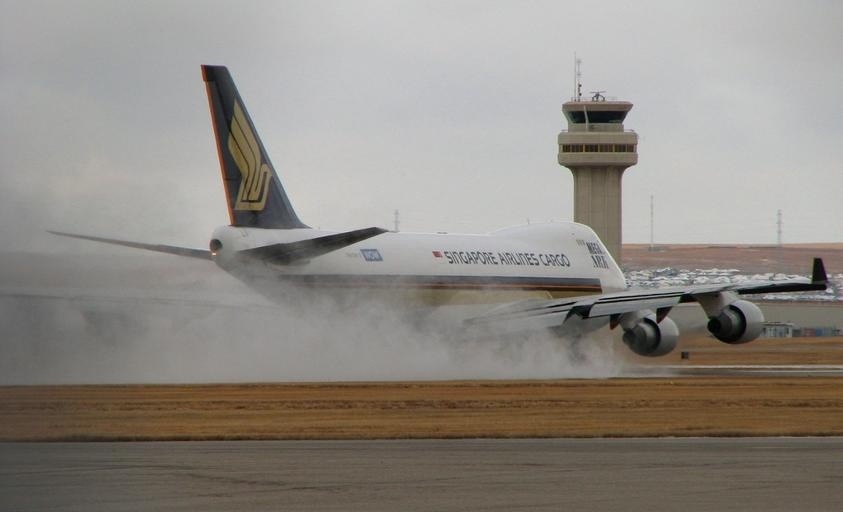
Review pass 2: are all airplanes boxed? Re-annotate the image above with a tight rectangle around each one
[43,62,830,358]
